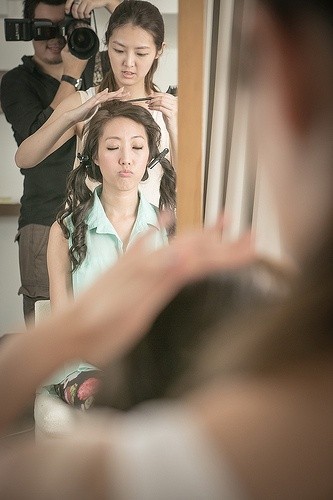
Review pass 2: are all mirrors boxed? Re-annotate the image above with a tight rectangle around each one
[0,0,208,446]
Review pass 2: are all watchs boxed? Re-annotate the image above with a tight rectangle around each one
[62,75,82,91]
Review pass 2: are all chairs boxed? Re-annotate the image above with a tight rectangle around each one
[34,300,83,443]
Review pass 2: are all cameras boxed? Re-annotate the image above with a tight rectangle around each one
[56,13,100,59]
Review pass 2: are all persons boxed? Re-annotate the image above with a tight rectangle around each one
[0,0,333,500]
[0,0,122,331]
[47,100,176,410]
[16,0,178,211]
